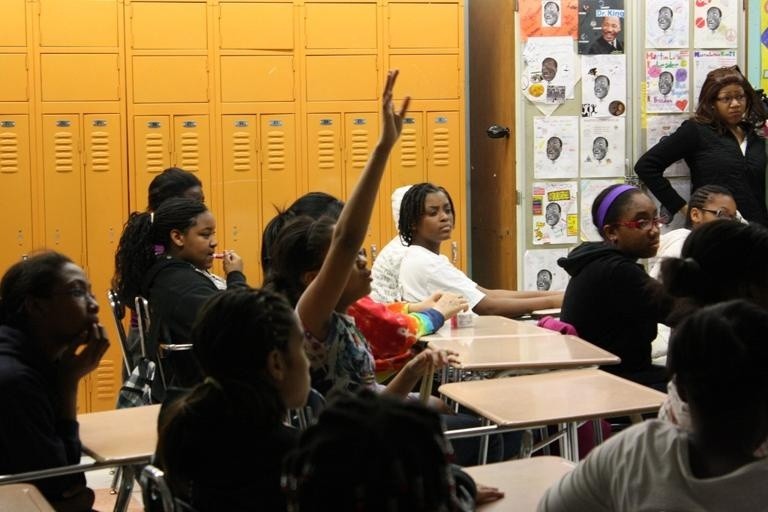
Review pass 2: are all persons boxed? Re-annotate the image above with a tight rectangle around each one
[0,249,111,512]
[363,185,411,304]
[634,64,768,227]
[588,16,623,54]
[120,167,204,384]
[397,183,566,318]
[531,300,767,511]
[557,184,710,425]
[269,71,462,421]
[144,290,506,511]
[260,385,477,512]
[660,219,767,315]
[645,184,737,282]
[109,195,251,404]
[261,191,469,386]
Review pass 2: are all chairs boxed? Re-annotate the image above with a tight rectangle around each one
[135,298,152,358]
[289,387,329,429]
[107,289,131,375]
[139,465,175,511]
[157,343,194,395]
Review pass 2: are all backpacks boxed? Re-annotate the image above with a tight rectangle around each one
[116,309,204,408]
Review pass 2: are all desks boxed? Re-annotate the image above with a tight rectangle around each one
[436,369,668,461]
[401,314,563,345]
[426,335,621,417]
[1,482,56,512]
[76,403,162,512]
[459,454,582,512]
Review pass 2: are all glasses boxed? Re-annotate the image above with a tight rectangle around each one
[717,94,746,103]
[616,214,669,231]
[702,208,741,223]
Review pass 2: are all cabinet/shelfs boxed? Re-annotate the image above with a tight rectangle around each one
[301,0,384,274]
[129,0,214,230]
[215,0,301,288]
[383,2,467,271]
[32,1,128,410]
[1,1,35,410]
